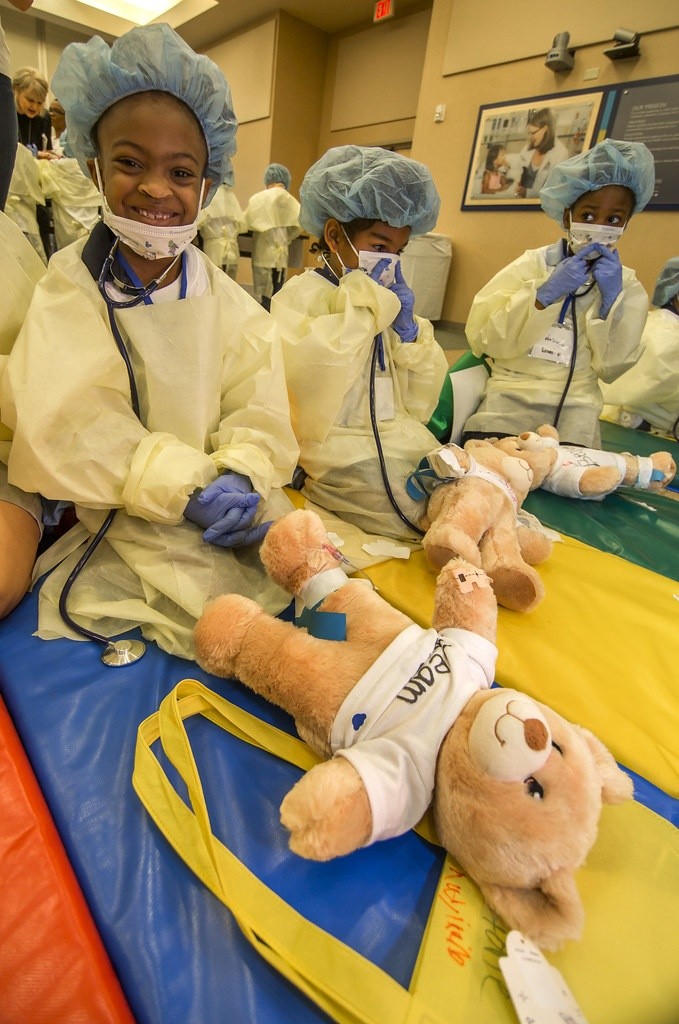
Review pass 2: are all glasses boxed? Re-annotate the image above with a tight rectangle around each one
[48,107,65,115]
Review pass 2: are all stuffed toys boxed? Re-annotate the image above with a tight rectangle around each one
[195,509,633,951]
[484,424,676,500]
[421,439,553,613]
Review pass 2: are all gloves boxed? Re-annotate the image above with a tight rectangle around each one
[182,469,275,547]
[345,259,392,286]
[534,241,599,308]
[383,261,419,342]
[591,244,623,319]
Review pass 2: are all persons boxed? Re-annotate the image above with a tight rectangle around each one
[0,21,301,621]
[480,108,570,198]
[460,137,658,449]
[598,254,679,443]
[268,144,540,543]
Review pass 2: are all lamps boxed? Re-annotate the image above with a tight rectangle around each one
[603,28,641,58]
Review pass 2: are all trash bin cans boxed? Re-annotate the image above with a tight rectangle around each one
[400,234,453,324]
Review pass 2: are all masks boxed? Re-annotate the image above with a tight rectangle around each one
[92,160,205,262]
[565,211,628,261]
[332,223,401,288]
